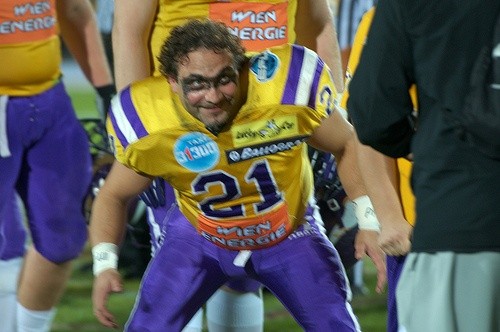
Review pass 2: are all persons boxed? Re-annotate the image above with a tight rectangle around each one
[91,20,388,332]
[347,0,500,332]
[0,0,116,332]
[111,0,345,332]
[341,5,417,331]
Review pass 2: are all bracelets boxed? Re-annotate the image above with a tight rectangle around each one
[351,194,382,233]
[90,241,119,276]
[95,84,117,123]
